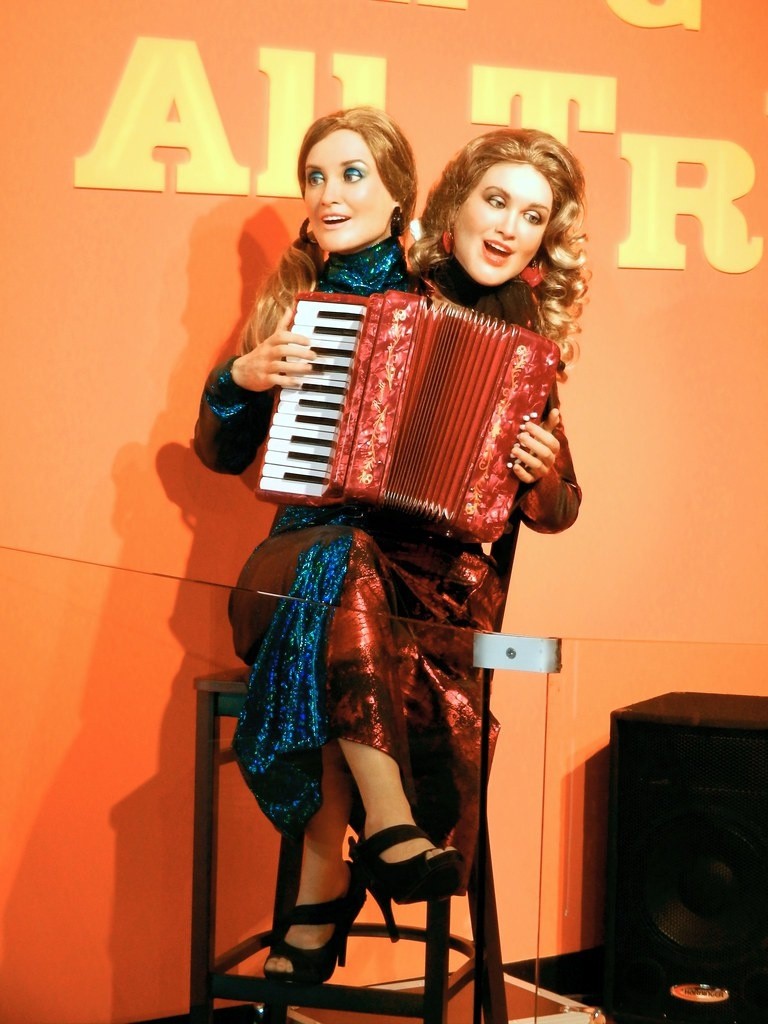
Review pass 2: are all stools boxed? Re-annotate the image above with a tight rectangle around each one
[188,667,509,1024]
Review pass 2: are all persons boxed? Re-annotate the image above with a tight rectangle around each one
[191,105,584,984]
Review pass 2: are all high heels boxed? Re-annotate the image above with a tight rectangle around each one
[262,861,366,993]
[356,825,466,943]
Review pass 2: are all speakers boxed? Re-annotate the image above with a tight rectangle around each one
[602,690,768,1024]
